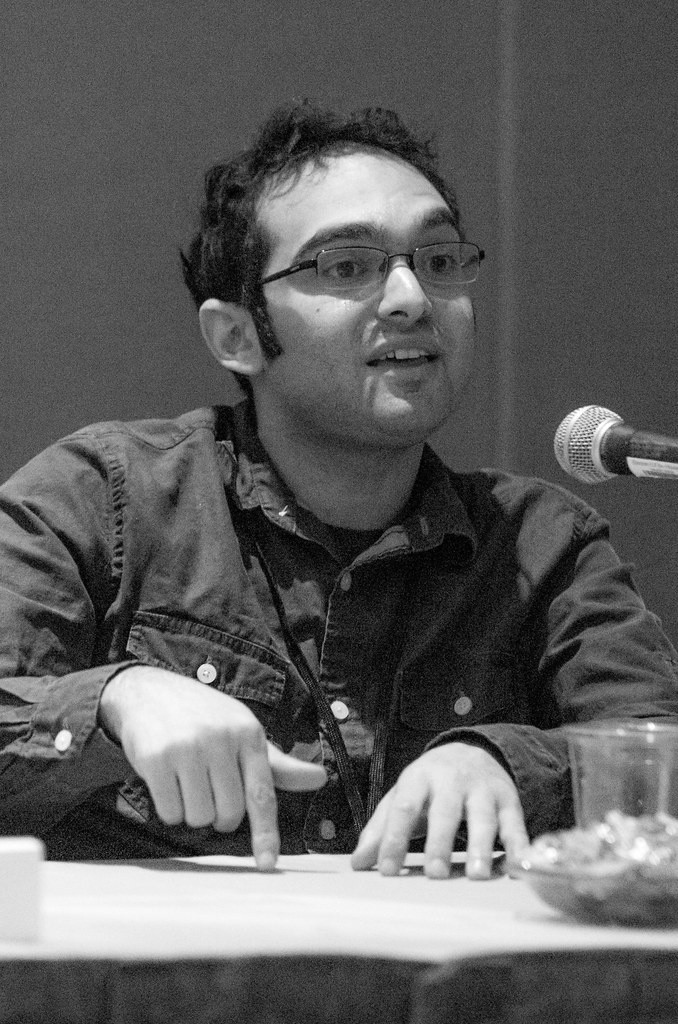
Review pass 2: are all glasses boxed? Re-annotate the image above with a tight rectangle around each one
[257,241,486,291]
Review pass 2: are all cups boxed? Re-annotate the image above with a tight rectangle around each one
[562,722,677,830]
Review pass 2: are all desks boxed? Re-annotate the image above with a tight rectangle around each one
[0,850,678,1024]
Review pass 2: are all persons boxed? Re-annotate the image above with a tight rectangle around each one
[0,99,678,879]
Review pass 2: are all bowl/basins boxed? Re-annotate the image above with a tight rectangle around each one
[502,860,678,929]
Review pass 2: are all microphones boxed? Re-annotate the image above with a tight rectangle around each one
[554,404,678,485]
